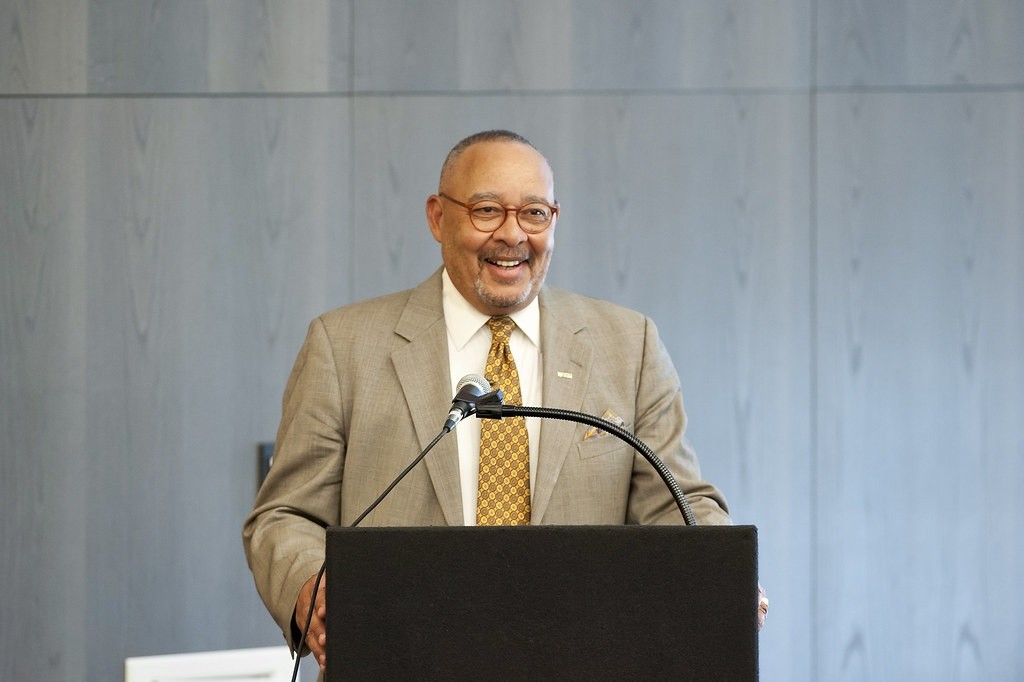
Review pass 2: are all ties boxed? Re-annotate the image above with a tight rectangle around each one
[475,316,532,526]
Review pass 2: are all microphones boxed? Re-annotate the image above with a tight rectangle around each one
[442,373,493,433]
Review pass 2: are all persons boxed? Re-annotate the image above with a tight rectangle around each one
[242,130,769,682]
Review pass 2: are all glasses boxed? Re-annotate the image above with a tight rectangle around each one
[438,190,560,235]
